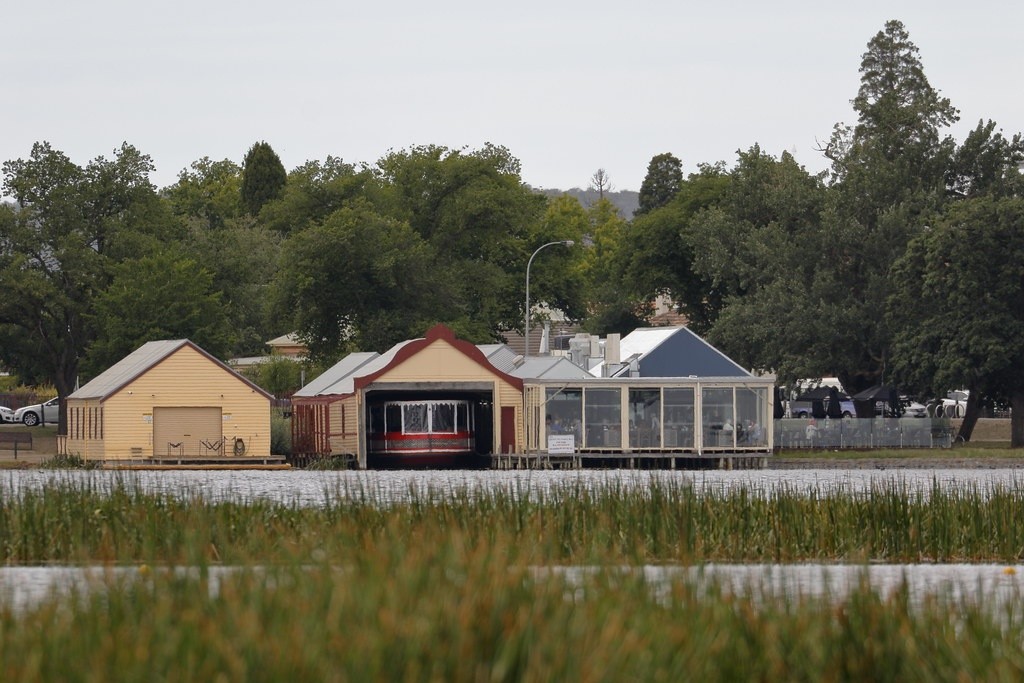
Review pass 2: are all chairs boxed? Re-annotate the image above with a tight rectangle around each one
[774,424,961,449]
[131,446,144,463]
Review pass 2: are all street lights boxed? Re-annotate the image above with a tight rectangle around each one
[524,239,575,357]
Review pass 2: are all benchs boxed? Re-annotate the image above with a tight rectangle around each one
[0,431,33,458]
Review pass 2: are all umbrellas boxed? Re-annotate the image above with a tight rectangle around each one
[773,384,904,419]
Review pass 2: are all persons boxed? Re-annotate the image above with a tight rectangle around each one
[546,411,762,447]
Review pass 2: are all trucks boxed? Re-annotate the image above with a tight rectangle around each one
[789,400,856,419]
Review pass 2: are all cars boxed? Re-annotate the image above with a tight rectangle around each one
[13,396,59,426]
[895,399,928,419]
[931,389,971,418]
[0,406,15,424]
